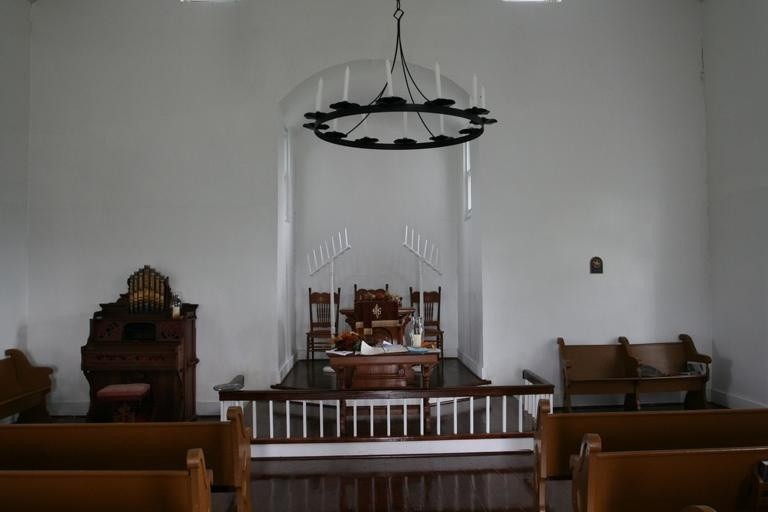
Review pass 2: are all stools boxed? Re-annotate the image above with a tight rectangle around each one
[96,382,150,422]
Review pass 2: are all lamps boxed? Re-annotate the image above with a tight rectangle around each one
[301,1,498,149]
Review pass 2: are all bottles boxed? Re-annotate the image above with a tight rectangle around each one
[408,316,425,348]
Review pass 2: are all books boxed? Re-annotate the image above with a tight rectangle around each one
[324,339,442,356]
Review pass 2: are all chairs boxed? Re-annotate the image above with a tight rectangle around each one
[409,286,444,377]
[305,287,340,368]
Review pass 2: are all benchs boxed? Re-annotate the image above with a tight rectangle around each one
[0,349,53,419]
[524,398,768,512]
[557,334,712,410]
[0,406,253,512]
